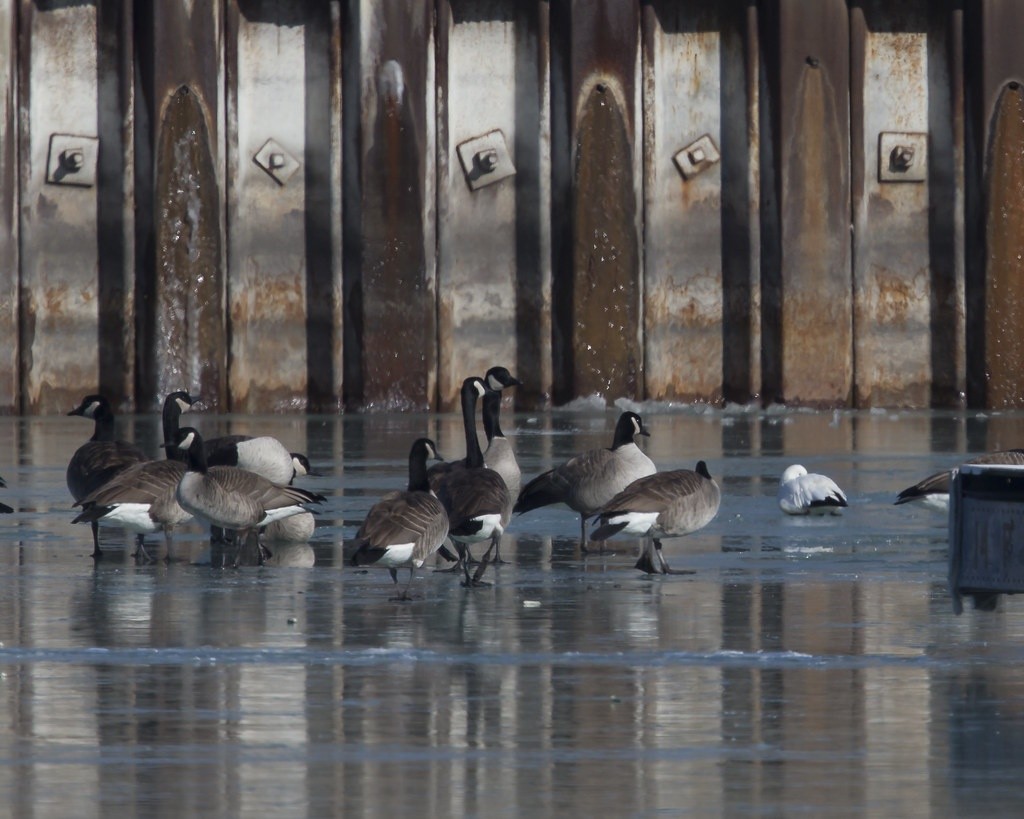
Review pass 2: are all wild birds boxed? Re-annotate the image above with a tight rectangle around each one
[348,437,449,602]
[202,436,322,488]
[70,391,205,564]
[67,395,151,558]
[512,412,658,554]
[893,450,1024,512]
[778,465,850,516]
[159,427,330,569]
[431,376,511,589]
[427,366,522,566]
[588,461,722,576]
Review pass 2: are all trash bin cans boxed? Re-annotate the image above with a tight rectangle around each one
[949,463,1024,594]
